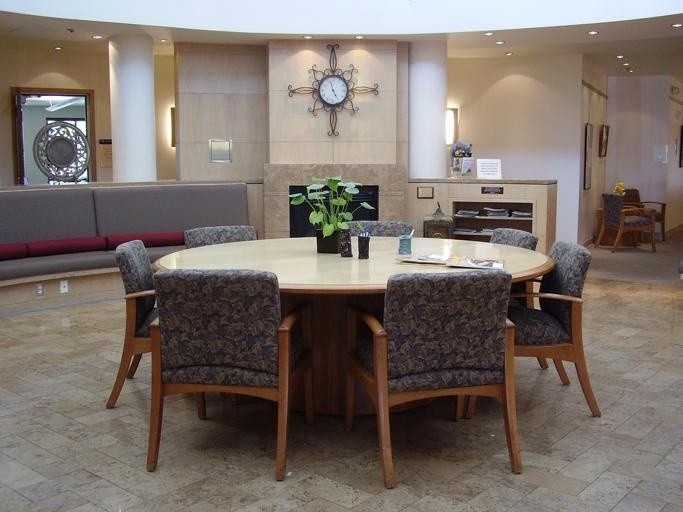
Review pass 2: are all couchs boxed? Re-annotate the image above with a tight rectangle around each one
[1,183,250,286]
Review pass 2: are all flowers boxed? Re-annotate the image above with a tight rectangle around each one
[613,182,626,198]
[449,142,471,157]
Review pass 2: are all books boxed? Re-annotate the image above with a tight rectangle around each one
[445,254,504,270]
[401,252,446,264]
[453,206,532,234]
[450,152,473,176]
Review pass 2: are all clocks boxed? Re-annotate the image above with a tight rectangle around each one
[288,44,379,137]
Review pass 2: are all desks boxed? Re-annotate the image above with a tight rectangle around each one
[410,179,557,254]
[152,236,555,417]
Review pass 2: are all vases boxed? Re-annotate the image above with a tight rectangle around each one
[451,158,468,179]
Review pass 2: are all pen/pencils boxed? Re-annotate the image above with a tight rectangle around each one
[360,231,370,238]
[409,229,415,238]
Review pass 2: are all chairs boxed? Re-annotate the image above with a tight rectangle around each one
[343,269,522,489]
[146,269,314,482]
[594,189,666,255]
[490,228,602,418]
[184,225,256,248]
[344,221,415,238]
[105,239,155,409]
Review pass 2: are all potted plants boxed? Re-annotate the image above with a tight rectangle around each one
[287,175,377,253]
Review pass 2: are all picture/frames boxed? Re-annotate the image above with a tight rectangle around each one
[584,124,593,190]
[599,124,609,157]
